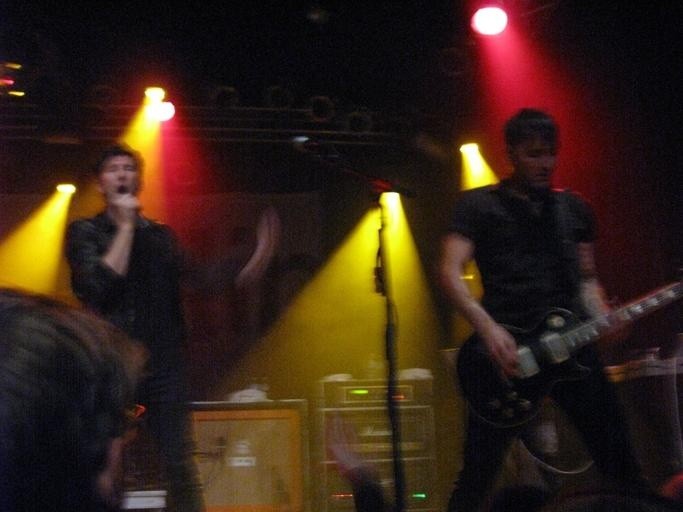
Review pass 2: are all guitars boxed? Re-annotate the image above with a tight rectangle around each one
[456,280,683,426]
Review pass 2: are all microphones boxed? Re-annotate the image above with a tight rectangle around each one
[119,184,158,239]
[337,160,414,200]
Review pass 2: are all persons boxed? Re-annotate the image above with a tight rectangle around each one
[64,148,283,511]
[325,413,544,510]
[0,285,148,512]
[436,109,659,509]
